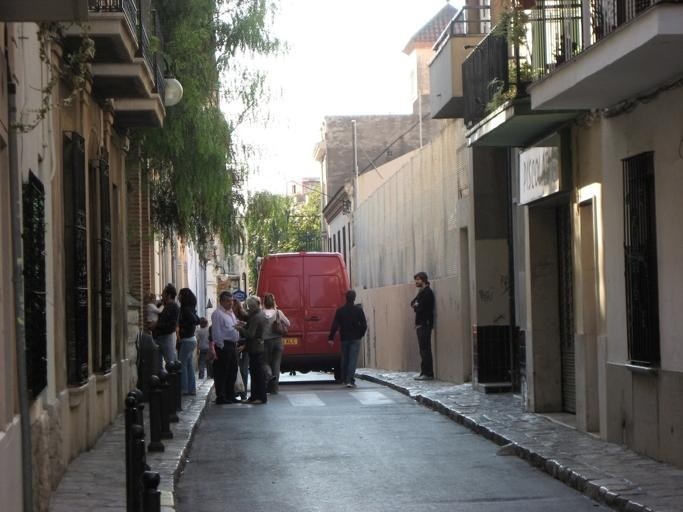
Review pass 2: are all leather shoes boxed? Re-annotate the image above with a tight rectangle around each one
[215,397,240,405]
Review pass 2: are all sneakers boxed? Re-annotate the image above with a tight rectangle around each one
[234,365,246,394]
[413,373,434,381]
[346,382,356,388]
[242,397,266,404]
[265,376,276,393]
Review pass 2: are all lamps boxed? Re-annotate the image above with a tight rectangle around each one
[155,53,183,108]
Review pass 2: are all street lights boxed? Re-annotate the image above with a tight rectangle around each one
[241,271,247,310]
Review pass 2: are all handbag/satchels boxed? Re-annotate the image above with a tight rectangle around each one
[271,310,289,337]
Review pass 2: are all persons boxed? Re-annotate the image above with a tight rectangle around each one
[142,283,292,405]
[328,290,367,387]
[411,272,435,380]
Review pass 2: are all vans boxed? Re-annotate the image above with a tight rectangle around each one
[250,250,352,381]
